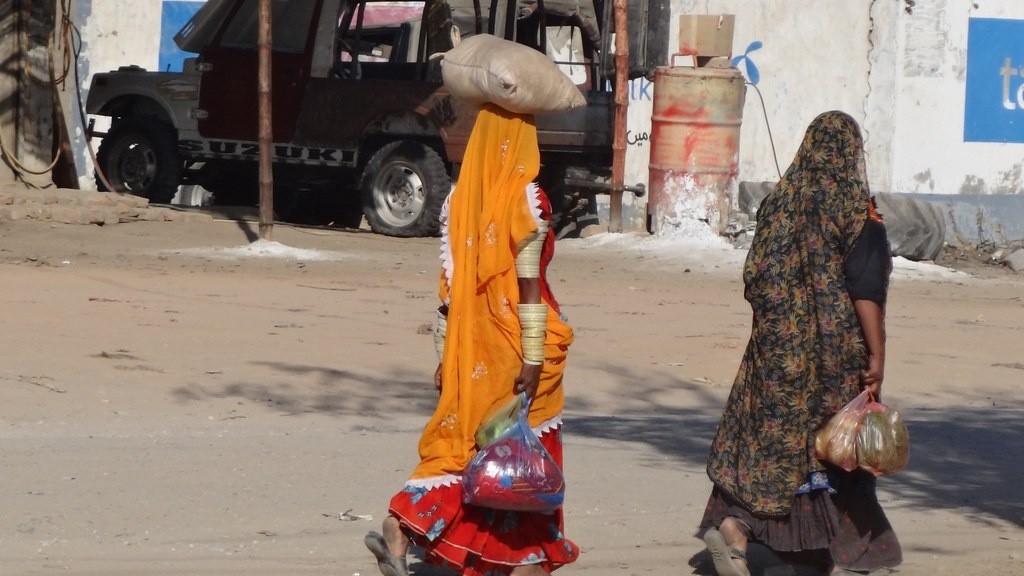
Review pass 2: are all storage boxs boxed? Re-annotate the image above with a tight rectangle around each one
[678,12,735,58]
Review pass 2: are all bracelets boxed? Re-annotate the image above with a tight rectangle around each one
[522,358,543,366]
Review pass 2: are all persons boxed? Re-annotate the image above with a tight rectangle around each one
[366,101,579,576]
[697,111,904,576]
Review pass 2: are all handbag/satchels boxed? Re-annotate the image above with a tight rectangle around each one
[856,388,910,476]
[815,387,869,472]
[475,393,523,447]
[461,393,566,513]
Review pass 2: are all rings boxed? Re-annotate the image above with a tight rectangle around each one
[865,371,870,378]
[516,384,526,391]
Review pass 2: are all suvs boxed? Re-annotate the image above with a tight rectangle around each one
[86,1,615,240]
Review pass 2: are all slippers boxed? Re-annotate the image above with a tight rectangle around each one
[705,529,751,576]
[363,531,409,576]
[831,570,889,576]
